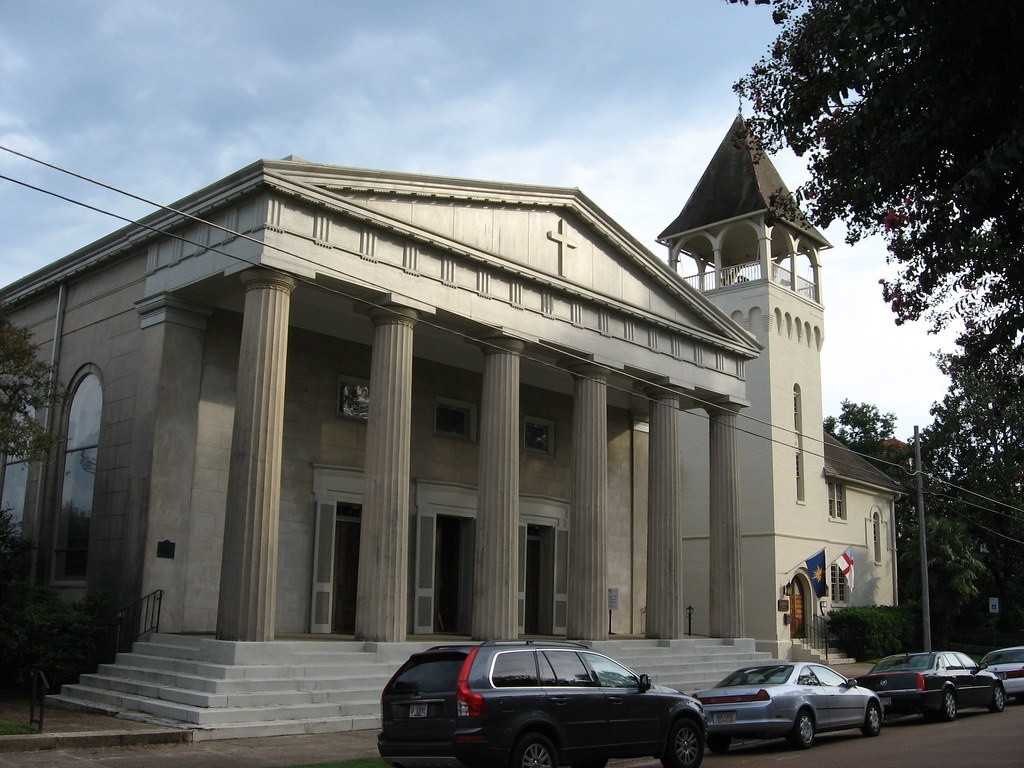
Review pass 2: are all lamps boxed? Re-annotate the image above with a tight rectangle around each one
[783,580,794,597]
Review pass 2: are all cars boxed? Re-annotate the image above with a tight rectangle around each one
[979,646,1024,704]
[854,650,1007,721]
[691,662,886,753]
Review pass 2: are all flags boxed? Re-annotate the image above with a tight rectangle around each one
[836,547,855,590]
[804,548,828,599]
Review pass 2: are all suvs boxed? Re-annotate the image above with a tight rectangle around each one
[376,638,709,768]
